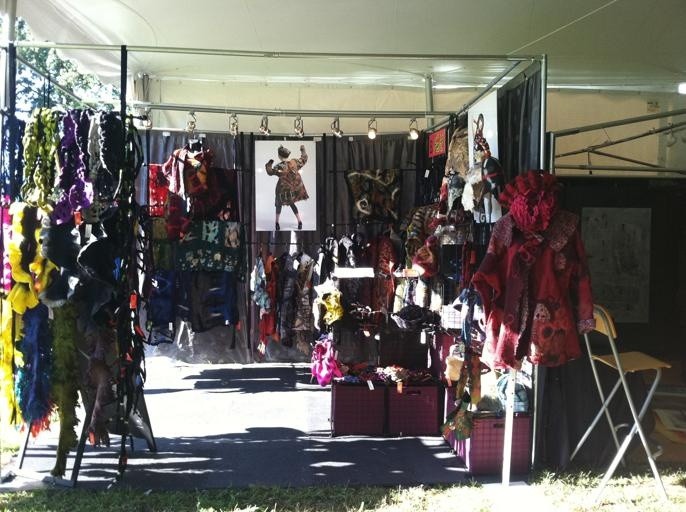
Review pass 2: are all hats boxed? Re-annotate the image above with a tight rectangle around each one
[279,146,291,157]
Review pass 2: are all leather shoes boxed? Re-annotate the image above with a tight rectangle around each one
[275,223,280,230]
[298,221,302,229]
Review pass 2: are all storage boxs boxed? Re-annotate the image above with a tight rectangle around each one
[330,379,532,476]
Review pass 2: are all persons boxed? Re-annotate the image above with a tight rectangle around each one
[266,144,310,230]
[472,114,491,164]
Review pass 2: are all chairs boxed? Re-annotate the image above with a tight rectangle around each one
[570,304,670,489]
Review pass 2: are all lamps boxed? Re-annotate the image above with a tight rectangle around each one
[133,102,420,142]
